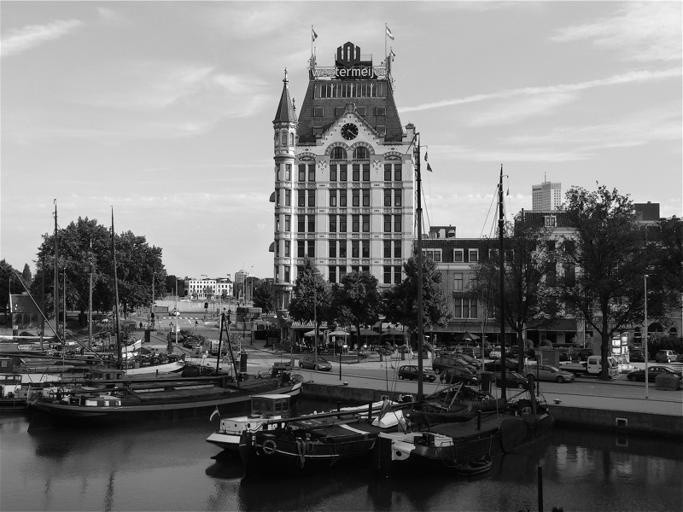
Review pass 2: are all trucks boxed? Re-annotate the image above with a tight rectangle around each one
[559,355,619,379]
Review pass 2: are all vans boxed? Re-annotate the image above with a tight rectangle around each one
[523,363,576,383]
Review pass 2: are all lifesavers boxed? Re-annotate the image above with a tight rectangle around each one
[261,438,277,455]
[108,344,115,350]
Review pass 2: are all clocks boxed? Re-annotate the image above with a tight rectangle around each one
[340,123,359,141]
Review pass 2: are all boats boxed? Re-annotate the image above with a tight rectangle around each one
[207,395,407,457]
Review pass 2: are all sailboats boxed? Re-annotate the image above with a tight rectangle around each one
[1,198,304,416]
[377,164,554,479]
[237,121,512,477]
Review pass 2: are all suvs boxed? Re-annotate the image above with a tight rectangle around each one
[398,364,437,383]
[297,356,333,372]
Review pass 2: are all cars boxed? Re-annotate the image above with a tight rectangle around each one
[431,341,524,388]
[656,350,682,363]
[631,349,650,362]
[627,365,681,383]
